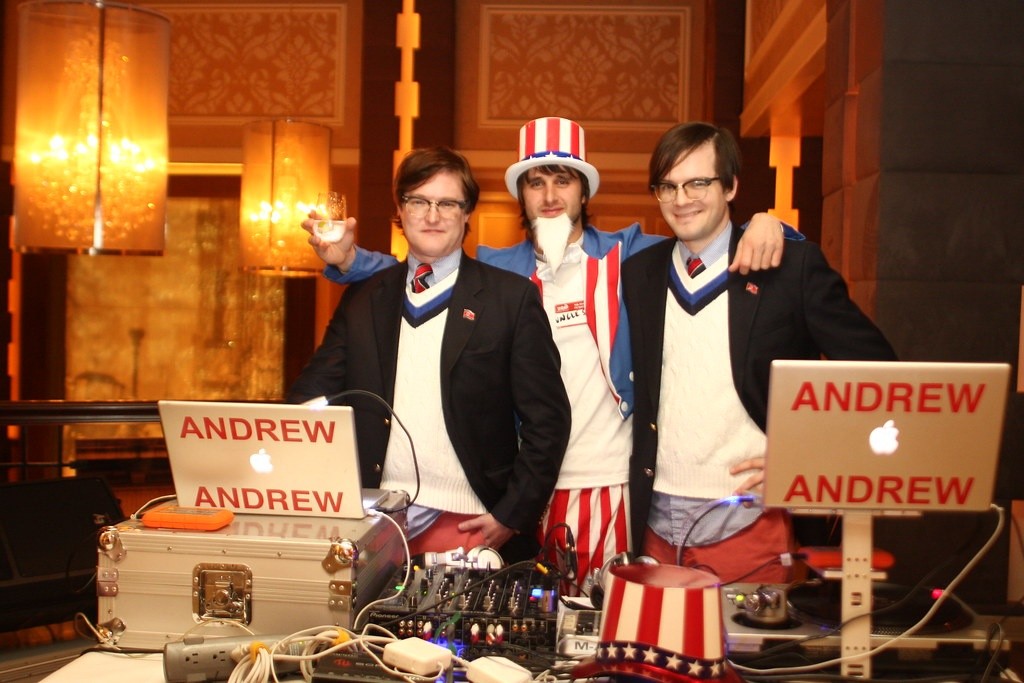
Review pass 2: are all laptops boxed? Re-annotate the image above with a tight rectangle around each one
[740,359,1012,512]
[157,398,396,522]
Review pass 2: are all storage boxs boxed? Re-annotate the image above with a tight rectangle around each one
[96,488,409,652]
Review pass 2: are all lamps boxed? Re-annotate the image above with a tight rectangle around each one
[241,1,333,275]
[13,1,171,257]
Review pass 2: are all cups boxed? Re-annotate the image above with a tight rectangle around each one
[312,192,347,242]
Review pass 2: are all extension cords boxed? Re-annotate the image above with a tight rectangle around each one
[166,634,313,683]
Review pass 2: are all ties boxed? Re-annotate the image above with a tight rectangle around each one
[410,263,433,293]
[686,256,707,279]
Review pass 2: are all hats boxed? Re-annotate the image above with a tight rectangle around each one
[504,117,600,200]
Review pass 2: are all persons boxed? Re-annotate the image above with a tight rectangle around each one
[619,121,900,586]
[300,116,787,599]
[281,143,574,564]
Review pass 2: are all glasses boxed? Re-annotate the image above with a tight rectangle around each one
[651,176,721,204]
[401,196,467,220]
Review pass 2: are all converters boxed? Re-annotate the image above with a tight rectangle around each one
[467,655,533,683]
[383,636,452,676]
